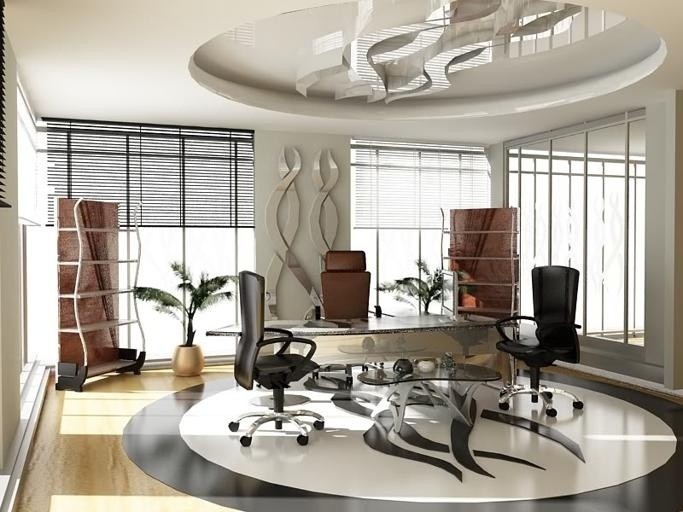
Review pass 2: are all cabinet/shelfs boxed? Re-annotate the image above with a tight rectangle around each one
[441,208,520,324]
[55,196,146,393]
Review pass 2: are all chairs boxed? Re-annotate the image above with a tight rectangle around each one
[496,265,584,417]
[313,250,383,387]
[229,271,324,447]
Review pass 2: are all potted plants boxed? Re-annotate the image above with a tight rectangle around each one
[131,262,239,377]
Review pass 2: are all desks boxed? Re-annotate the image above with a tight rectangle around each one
[206,314,519,407]
